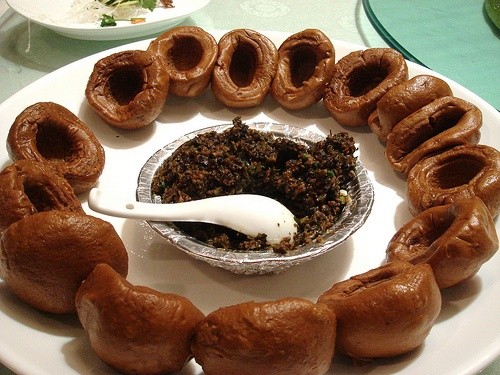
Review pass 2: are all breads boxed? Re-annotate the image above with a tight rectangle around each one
[0,25,500,375]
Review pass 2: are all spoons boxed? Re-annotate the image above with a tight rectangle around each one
[88,187,299,249]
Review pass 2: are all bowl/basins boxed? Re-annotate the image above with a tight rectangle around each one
[136,121,375,275]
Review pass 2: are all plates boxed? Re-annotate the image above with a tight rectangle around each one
[0,30,500,375]
[5,0,210,42]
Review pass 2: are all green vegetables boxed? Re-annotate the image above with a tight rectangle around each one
[100,0,158,27]
